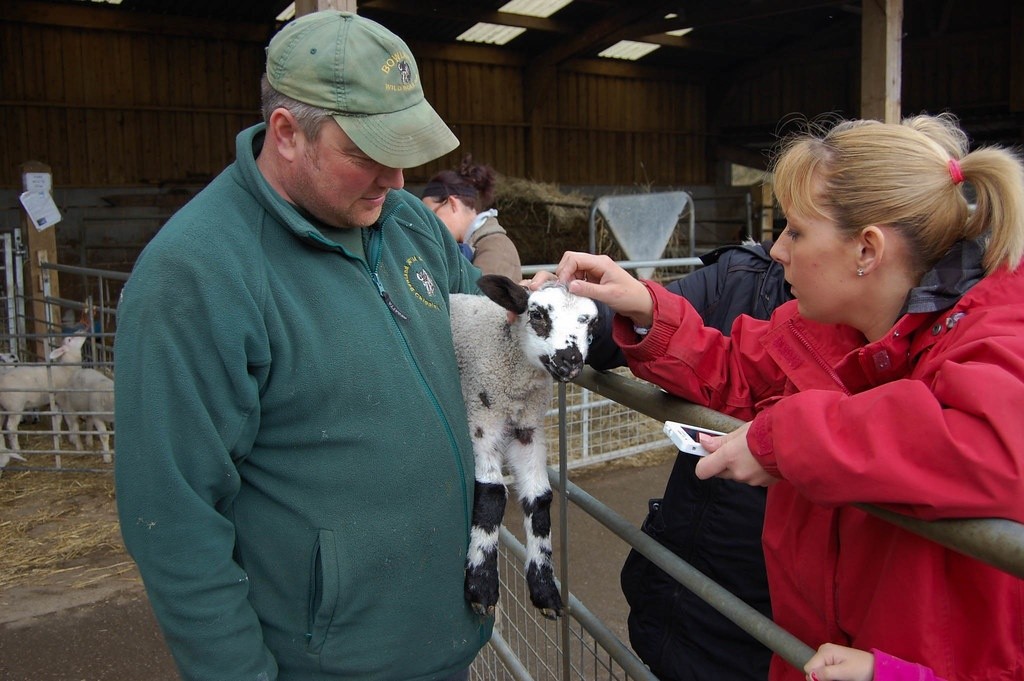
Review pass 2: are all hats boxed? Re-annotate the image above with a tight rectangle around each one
[267,10,461,169]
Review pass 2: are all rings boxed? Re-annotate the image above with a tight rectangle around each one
[583,269,587,281]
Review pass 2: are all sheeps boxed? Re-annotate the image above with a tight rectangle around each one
[0,320,114,476]
[447,272,600,622]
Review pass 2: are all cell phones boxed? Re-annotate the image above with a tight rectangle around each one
[662,420,730,457]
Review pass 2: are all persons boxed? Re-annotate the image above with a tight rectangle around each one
[552,124,1024,681]
[506,113,967,681]
[423,155,522,294]
[113,9,495,681]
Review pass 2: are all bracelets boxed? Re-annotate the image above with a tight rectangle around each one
[633,324,648,335]
